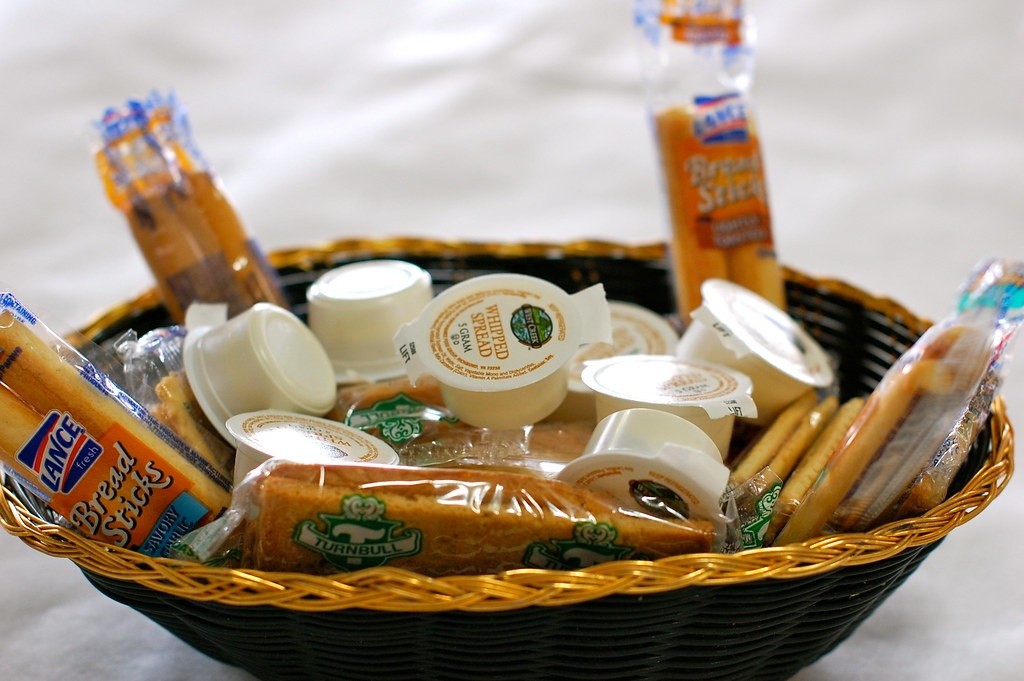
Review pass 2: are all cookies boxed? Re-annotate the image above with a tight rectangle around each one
[720,395,866,546]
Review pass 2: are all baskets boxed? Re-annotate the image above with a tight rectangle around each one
[0,235,1016,681]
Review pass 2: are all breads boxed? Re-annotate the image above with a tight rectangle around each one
[0,305,235,564]
[654,99,787,321]
[231,462,716,576]
[149,368,603,467]
[776,324,990,546]
[123,171,290,323]
